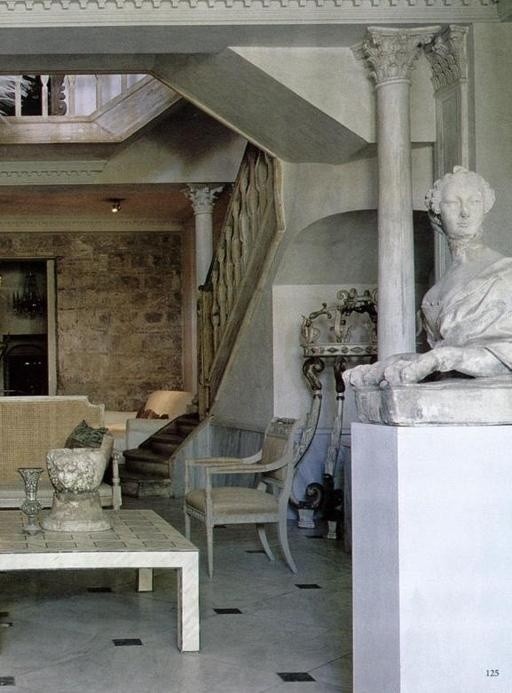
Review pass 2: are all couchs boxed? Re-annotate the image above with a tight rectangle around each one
[0,392,124,510]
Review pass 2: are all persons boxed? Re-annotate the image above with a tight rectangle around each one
[342,165,512,386]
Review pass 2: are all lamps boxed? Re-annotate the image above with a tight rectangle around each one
[11,267,46,318]
[110,201,123,214]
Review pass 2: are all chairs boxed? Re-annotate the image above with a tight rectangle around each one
[181,414,301,580]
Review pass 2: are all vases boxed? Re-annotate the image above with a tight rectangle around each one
[15,465,45,534]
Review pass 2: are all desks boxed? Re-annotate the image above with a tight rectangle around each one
[0,505,202,655]
